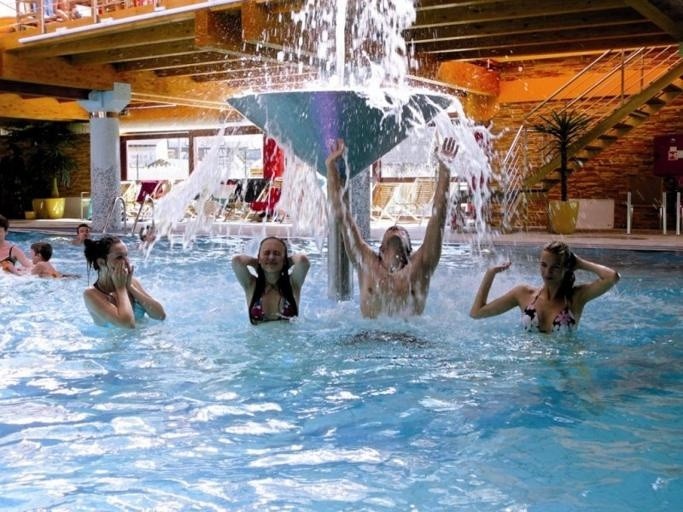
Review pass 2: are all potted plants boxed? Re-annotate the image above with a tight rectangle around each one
[22,128,82,219]
[534,102,596,234]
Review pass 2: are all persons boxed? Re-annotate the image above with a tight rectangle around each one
[84,237,165,329]
[232,236,310,325]
[73,223,89,244]
[470,241,621,332]
[0,215,56,276]
[325,137,459,319]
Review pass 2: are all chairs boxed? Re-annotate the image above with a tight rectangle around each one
[368,176,437,226]
